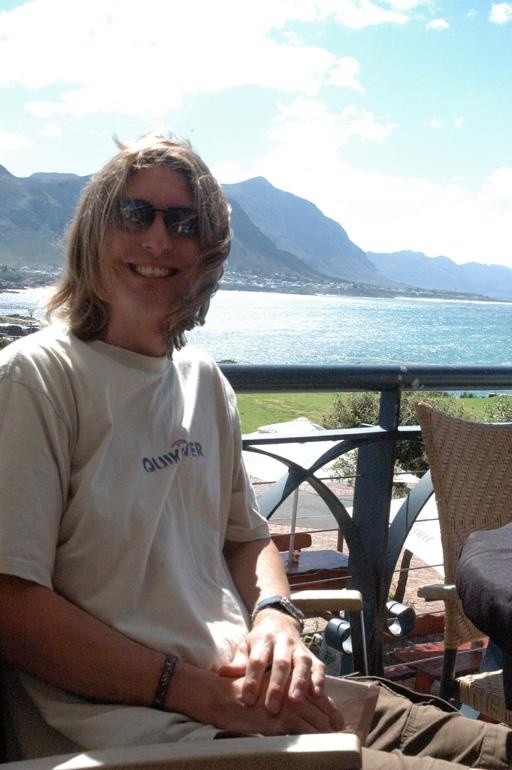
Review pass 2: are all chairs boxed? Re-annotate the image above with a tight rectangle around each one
[418,401,511,727]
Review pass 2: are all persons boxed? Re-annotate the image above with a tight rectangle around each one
[0,127,512,769]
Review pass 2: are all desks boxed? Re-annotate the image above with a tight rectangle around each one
[280,550,348,575]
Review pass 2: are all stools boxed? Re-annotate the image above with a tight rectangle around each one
[390,638,490,693]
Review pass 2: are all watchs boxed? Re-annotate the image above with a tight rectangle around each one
[250,595,305,627]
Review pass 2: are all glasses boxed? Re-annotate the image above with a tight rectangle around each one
[115,198,196,238]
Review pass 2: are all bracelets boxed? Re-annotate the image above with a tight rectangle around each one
[151,650,179,712]
